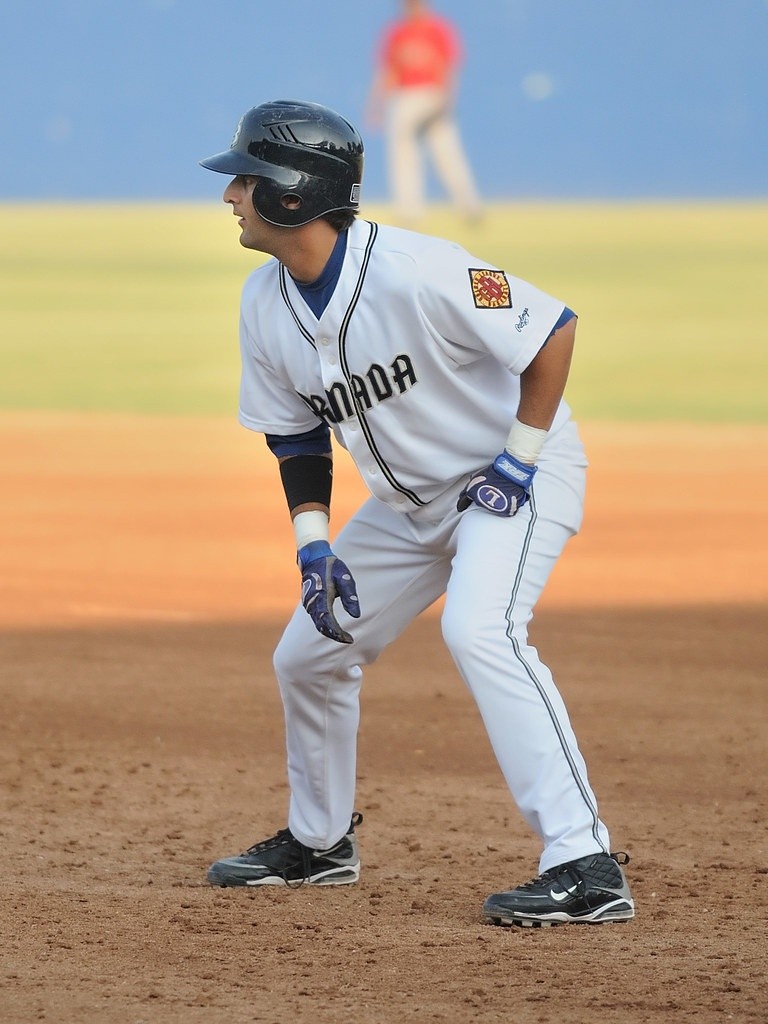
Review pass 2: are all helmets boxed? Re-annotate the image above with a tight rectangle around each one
[200,99,365,226]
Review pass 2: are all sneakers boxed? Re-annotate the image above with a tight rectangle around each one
[207,811,364,889]
[482,852,636,927]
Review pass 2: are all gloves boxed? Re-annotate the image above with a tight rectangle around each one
[296,540,361,644]
[458,452,538,518]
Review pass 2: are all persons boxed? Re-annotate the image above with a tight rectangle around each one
[376,1,486,234]
[200,98,636,926]
